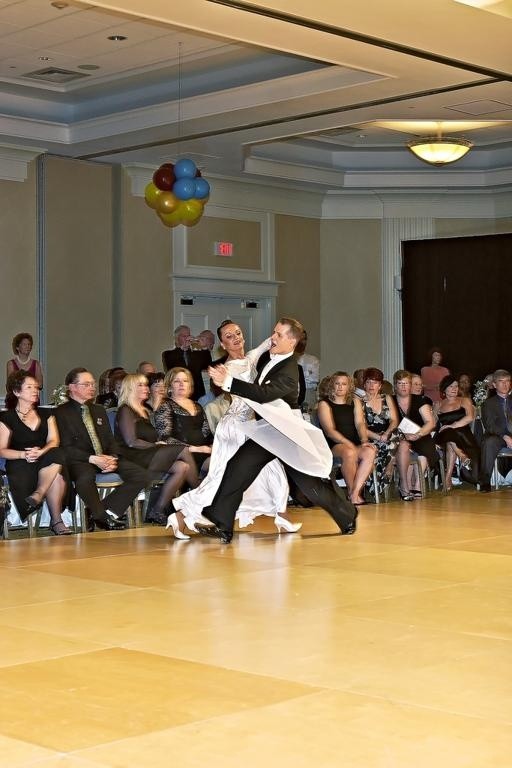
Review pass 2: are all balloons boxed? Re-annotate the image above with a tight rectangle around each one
[144,158,211,229]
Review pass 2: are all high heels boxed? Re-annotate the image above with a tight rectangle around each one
[165,513,191,539]
[462,458,470,471]
[274,516,303,534]
[48,521,72,535]
[25,492,42,511]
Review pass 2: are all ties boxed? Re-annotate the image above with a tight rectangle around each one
[80,405,103,456]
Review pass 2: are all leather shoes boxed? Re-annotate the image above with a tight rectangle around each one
[342,507,357,535]
[85,507,125,532]
[479,484,491,493]
[399,490,422,501]
[346,496,368,505]
[147,518,166,526]
[194,523,233,544]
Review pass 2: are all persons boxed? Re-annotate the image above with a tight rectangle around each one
[288,349,512,504]
[1,323,215,534]
[167,318,360,544]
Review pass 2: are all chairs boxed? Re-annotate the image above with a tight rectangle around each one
[0,407,512,539]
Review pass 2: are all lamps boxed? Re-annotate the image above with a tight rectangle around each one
[408,136,474,165]
